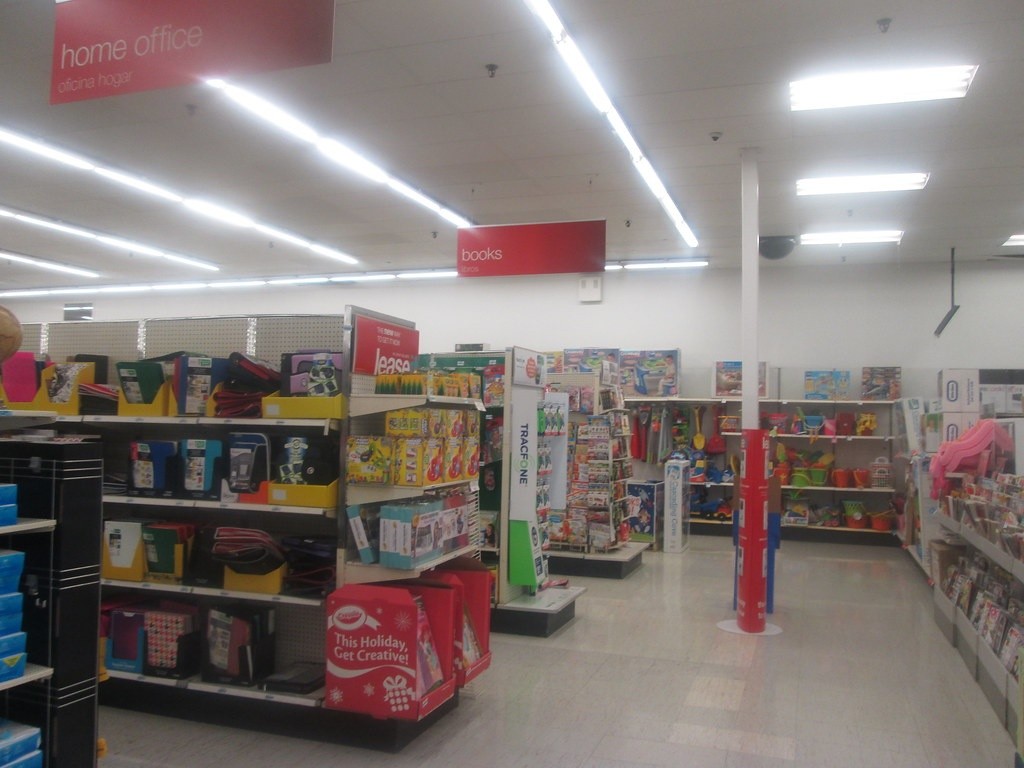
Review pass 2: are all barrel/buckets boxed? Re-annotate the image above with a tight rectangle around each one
[719,413,876,487]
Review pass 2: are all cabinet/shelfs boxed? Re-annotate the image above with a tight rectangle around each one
[343,394,482,585]
[624,398,896,533]
[0,412,56,768]
[933,510,1024,750]
[55,415,340,707]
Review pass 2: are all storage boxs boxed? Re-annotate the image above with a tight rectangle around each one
[804,370,850,400]
[380,495,468,570]
[346,502,384,564]
[626,480,665,543]
[346,408,480,487]
[0,483,17,505]
[0,631,35,659]
[860,367,902,399]
[0,652,36,683]
[375,373,482,399]
[0,750,42,768]
[541,348,681,398]
[0,593,32,637]
[0,549,25,596]
[664,459,691,555]
[895,368,1024,452]
[0,504,18,526]
[716,360,767,398]
[0,719,42,768]
